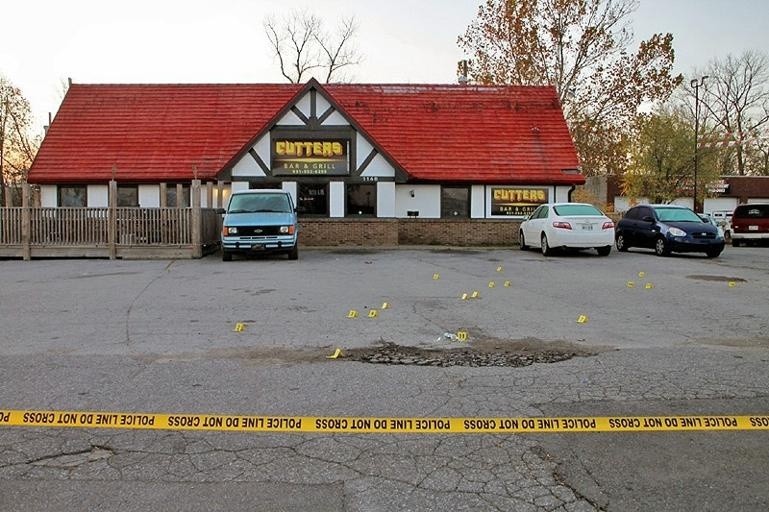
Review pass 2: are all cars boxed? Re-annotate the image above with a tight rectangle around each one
[615,199,768,255]
[213,183,305,261]
[516,201,615,256]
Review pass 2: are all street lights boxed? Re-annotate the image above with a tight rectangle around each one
[687,74,715,212]
